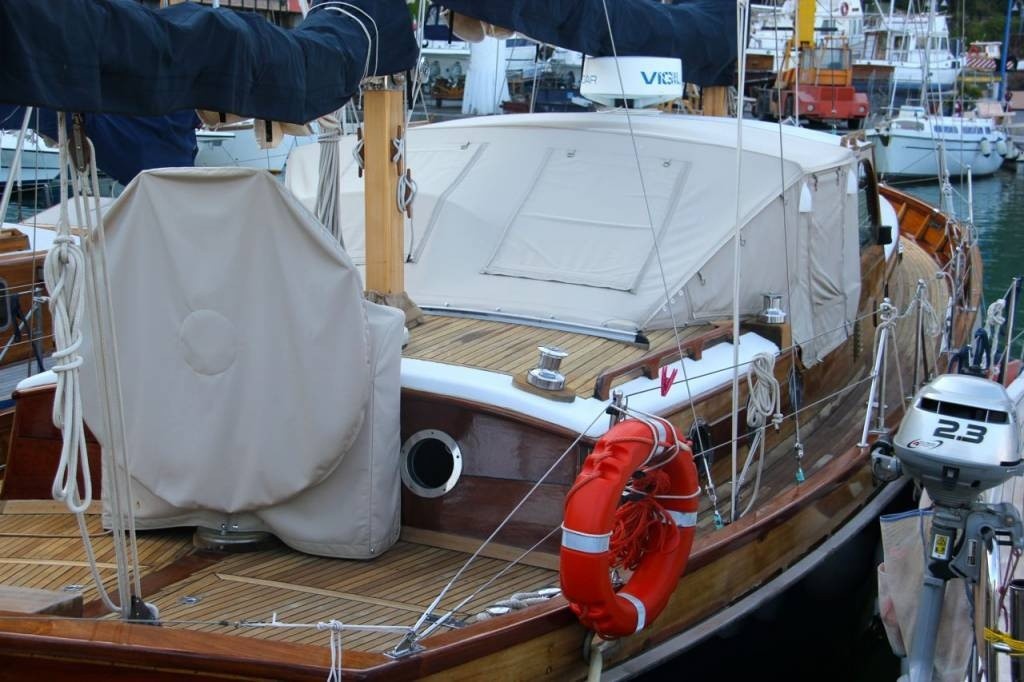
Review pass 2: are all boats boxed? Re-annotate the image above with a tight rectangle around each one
[1,0,1024,682]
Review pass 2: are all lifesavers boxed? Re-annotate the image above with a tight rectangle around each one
[560,418,703,638]
[969,46,978,57]
[841,2,849,16]
